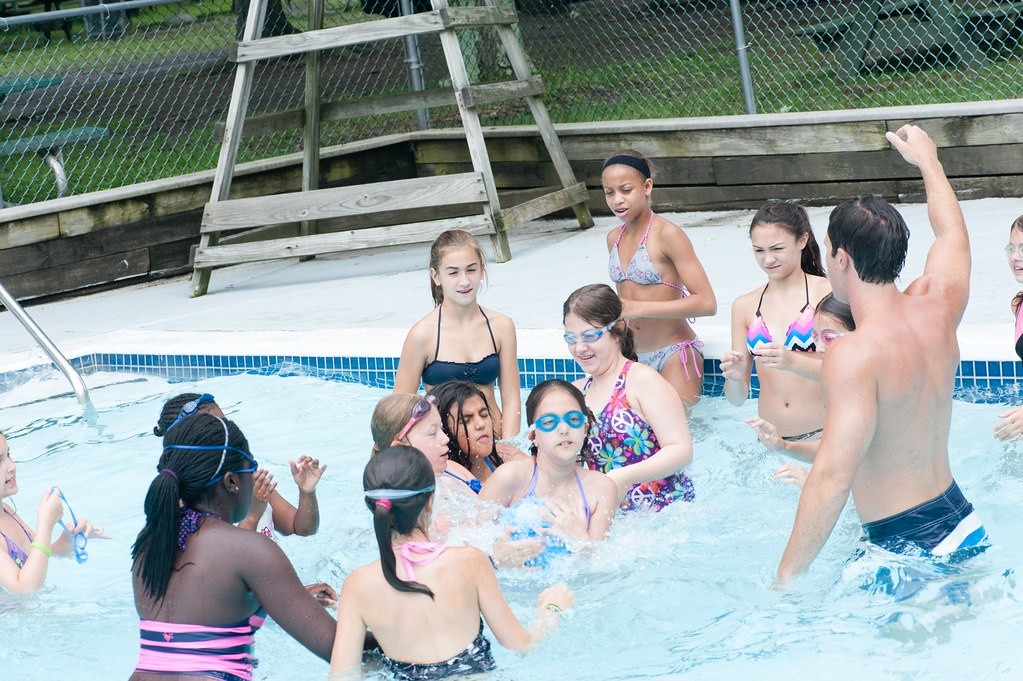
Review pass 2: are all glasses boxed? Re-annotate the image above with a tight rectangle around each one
[1002,244,1023,257]
[47,485,90,565]
[163,394,214,432]
[563,318,619,345]
[529,409,588,436]
[155,443,258,487]
[395,393,440,442]
[808,328,848,348]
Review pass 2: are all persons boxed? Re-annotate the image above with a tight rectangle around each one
[720,200,834,441]
[330,444,576,681]
[994,213,1023,445]
[0,432,113,595]
[153,394,328,536]
[744,291,856,487]
[370,378,617,572]
[602,148,718,418]
[775,125,997,628]
[127,413,384,681]
[563,285,696,516]
[391,229,522,440]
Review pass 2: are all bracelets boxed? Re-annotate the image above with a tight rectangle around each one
[32,541,52,557]
[543,604,563,614]
[775,439,788,452]
[489,556,499,571]
